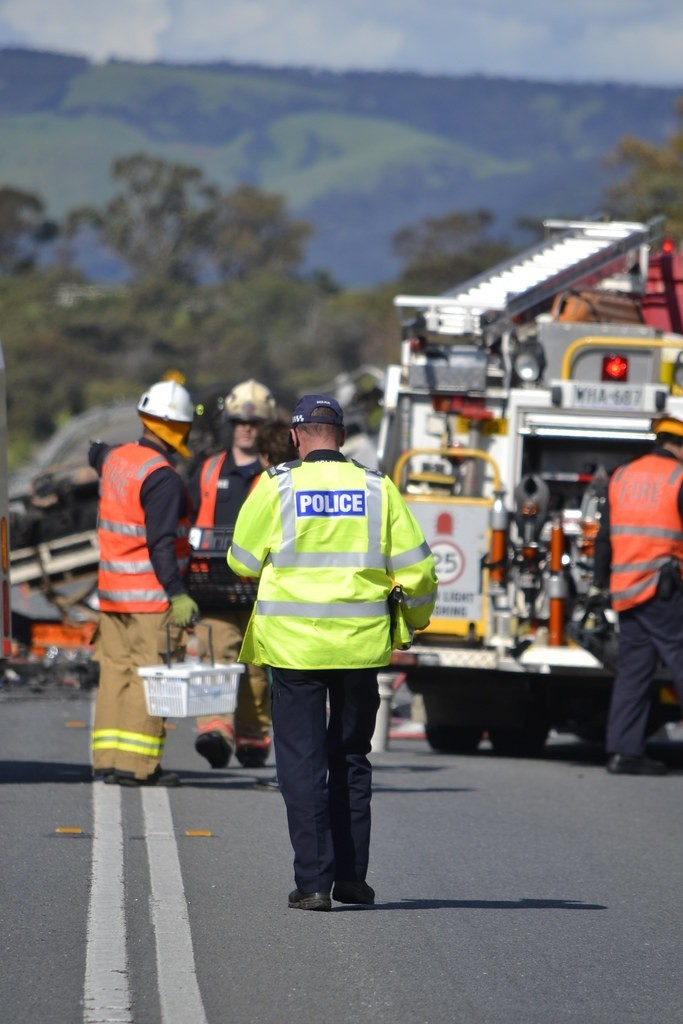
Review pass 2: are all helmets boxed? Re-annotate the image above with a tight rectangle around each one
[138,380,194,423]
[224,377,278,425]
[651,397,683,421]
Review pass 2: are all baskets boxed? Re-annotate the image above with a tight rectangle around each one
[183,526,261,614]
[136,621,246,718]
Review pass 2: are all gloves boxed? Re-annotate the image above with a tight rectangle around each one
[88,439,107,466]
[169,593,200,628]
[585,581,607,614]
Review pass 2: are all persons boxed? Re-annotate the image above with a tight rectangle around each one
[91,380,436,916]
[593,393,683,775]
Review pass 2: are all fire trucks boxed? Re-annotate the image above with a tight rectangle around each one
[368,211,683,757]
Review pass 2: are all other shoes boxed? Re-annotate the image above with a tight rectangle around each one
[117,772,180,787]
[195,730,232,769]
[607,750,668,776]
[102,772,117,783]
[333,878,376,905]
[235,746,266,768]
[287,887,332,911]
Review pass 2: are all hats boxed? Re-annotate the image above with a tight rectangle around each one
[288,395,344,444]
